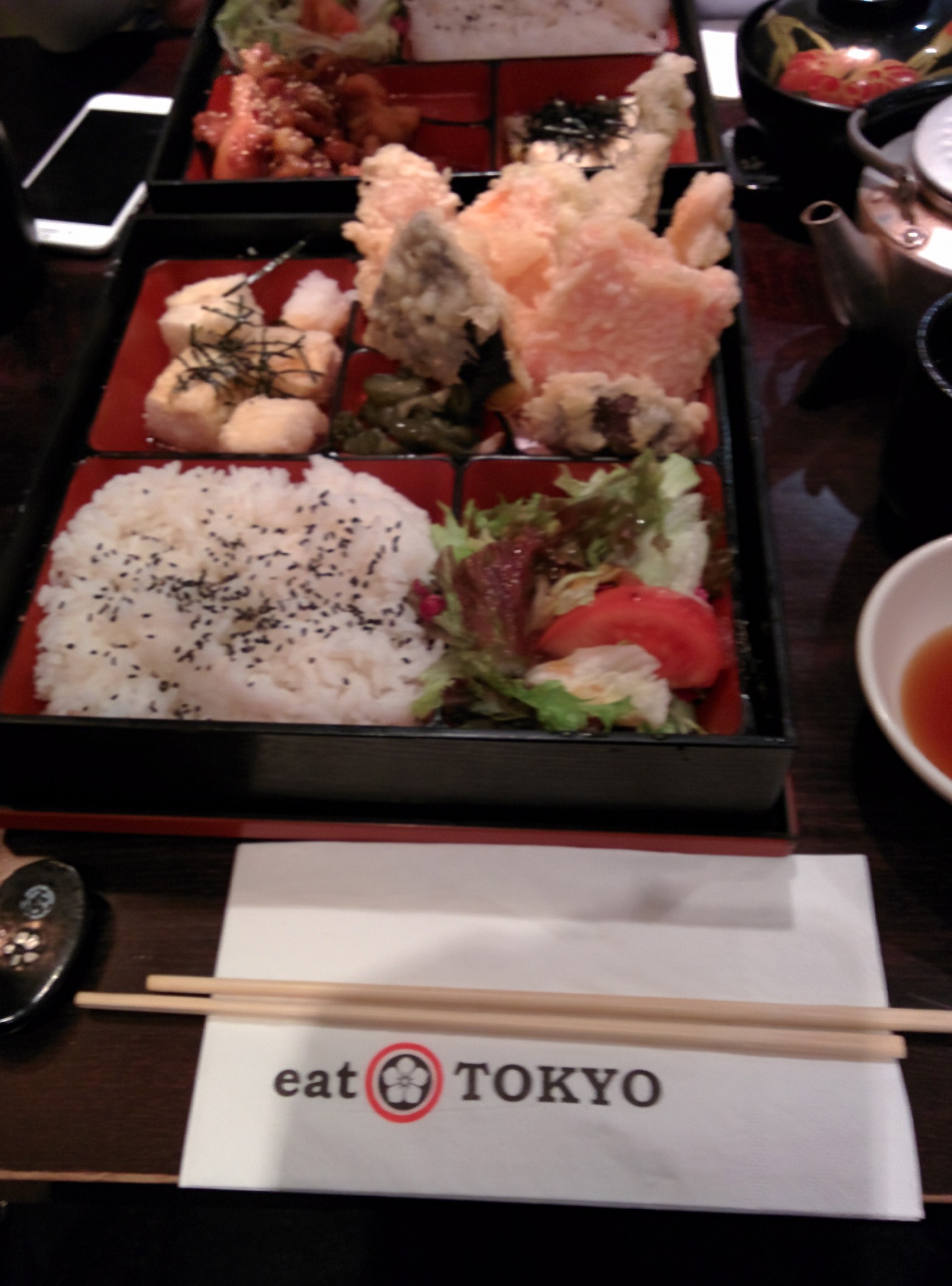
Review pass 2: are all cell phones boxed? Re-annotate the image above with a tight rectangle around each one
[19,92,174,254]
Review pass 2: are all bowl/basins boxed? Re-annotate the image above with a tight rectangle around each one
[855,536,952,802]
[1,254,799,848]
[912,290,952,414]
[737,0,952,171]
[144,0,733,264]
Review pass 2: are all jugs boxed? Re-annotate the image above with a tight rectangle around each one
[799,75,952,411]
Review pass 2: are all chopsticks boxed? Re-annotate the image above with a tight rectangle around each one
[70,975,951,1064]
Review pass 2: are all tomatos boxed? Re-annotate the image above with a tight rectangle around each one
[540,580,729,697]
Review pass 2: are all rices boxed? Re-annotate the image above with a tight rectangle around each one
[32,450,454,725]
